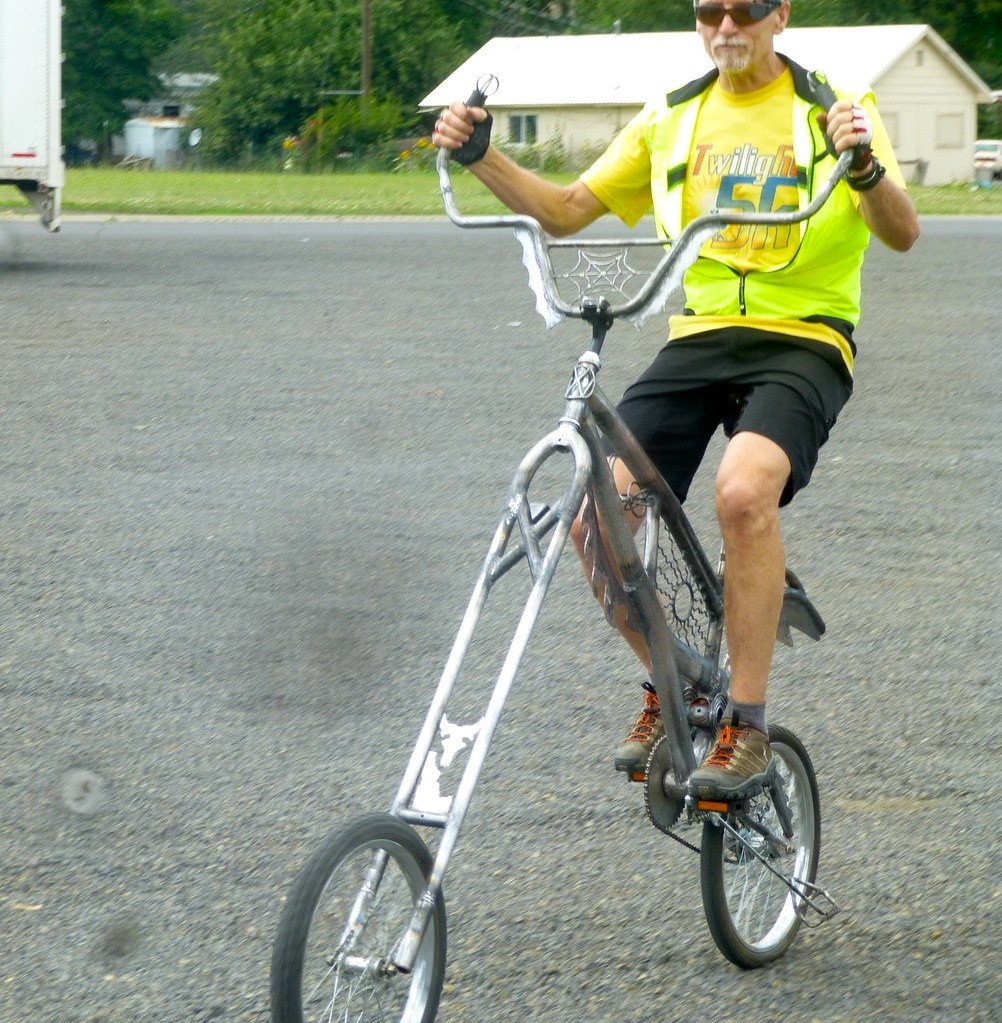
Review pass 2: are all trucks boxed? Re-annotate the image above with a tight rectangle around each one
[0,0,60,233]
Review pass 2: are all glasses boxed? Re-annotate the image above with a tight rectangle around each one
[694,0,781,25]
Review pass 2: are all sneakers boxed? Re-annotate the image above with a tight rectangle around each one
[684,710,776,801]
[615,681,665,768]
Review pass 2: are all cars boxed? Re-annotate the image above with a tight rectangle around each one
[972,139,1002,182]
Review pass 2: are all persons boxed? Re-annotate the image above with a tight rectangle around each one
[431,0,922,803]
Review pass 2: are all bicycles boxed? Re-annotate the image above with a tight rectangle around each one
[263,77,865,1022]
